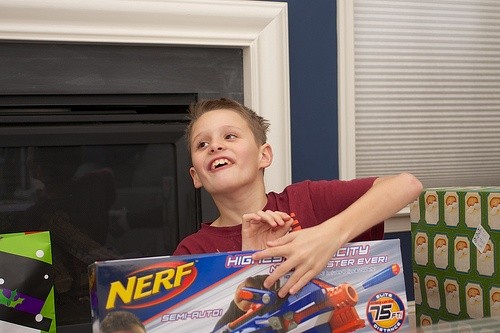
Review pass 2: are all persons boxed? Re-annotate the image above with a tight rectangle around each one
[171,96,424,297]
